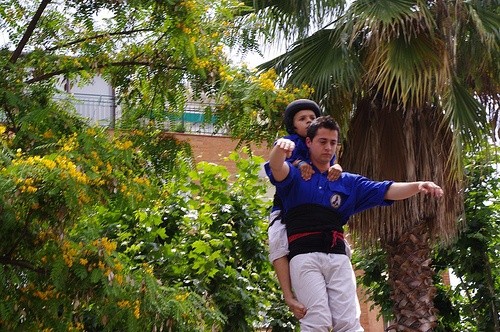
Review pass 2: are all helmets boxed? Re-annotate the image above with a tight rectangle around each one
[285,99,321,134]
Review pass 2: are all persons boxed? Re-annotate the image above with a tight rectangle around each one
[262,99,352,318]
[269,116,443,332]
